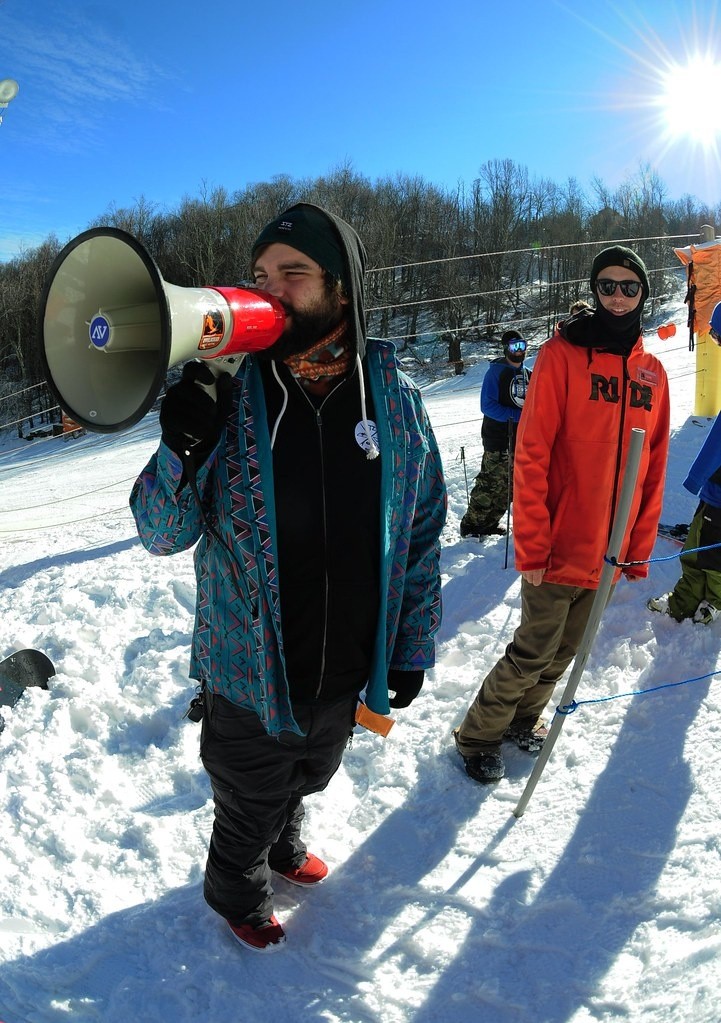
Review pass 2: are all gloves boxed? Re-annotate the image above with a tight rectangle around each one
[159,358,233,480]
[384,669,425,709]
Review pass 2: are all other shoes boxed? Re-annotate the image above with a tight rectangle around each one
[459,527,506,539]
[457,741,506,783]
[648,592,693,620]
[692,601,721,626]
[503,719,548,752]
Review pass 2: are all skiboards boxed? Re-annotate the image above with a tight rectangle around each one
[656,522,690,545]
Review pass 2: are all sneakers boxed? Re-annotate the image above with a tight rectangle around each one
[226,915,288,953]
[268,849,331,889]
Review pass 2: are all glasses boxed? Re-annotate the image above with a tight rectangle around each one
[507,339,527,353]
[595,277,645,298]
[708,328,721,346]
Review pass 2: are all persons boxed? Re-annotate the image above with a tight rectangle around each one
[450,336,464,374]
[129,203,447,955]
[452,246,670,781]
[460,331,533,538]
[647,302,721,626]
[569,302,591,316]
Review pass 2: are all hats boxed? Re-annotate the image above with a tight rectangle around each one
[710,301,721,338]
[501,331,525,363]
[589,246,651,302]
[252,207,352,287]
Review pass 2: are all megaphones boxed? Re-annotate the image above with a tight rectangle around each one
[39,227,286,435]
